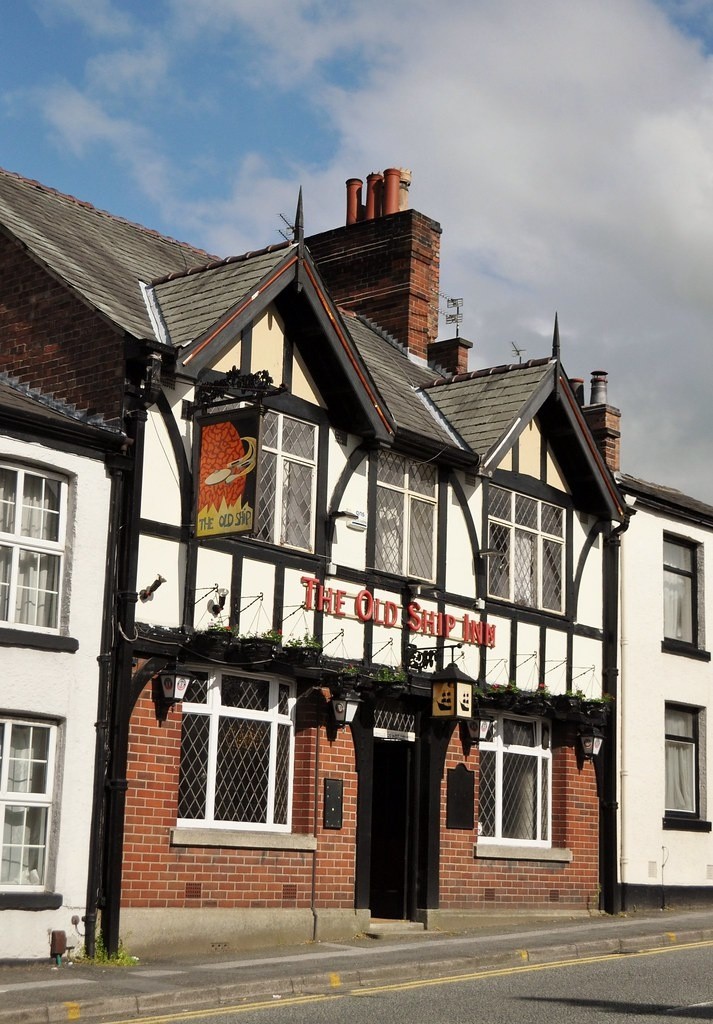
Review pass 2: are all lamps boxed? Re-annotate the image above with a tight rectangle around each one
[140,573,167,602]
[407,642,473,722]
[155,660,197,719]
[464,708,494,748]
[576,724,604,762]
[327,686,366,730]
[212,588,228,616]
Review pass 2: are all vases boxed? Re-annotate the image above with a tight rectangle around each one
[520,694,551,715]
[583,700,609,721]
[488,691,522,709]
[337,672,361,689]
[239,638,278,672]
[194,631,232,655]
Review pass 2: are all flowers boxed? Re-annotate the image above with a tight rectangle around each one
[196,619,241,637]
[338,663,362,676]
[523,683,553,699]
[583,692,617,704]
[475,679,522,699]
[237,626,283,642]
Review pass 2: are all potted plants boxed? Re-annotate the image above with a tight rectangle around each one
[369,663,407,702]
[551,688,586,717]
[283,629,322,669]
